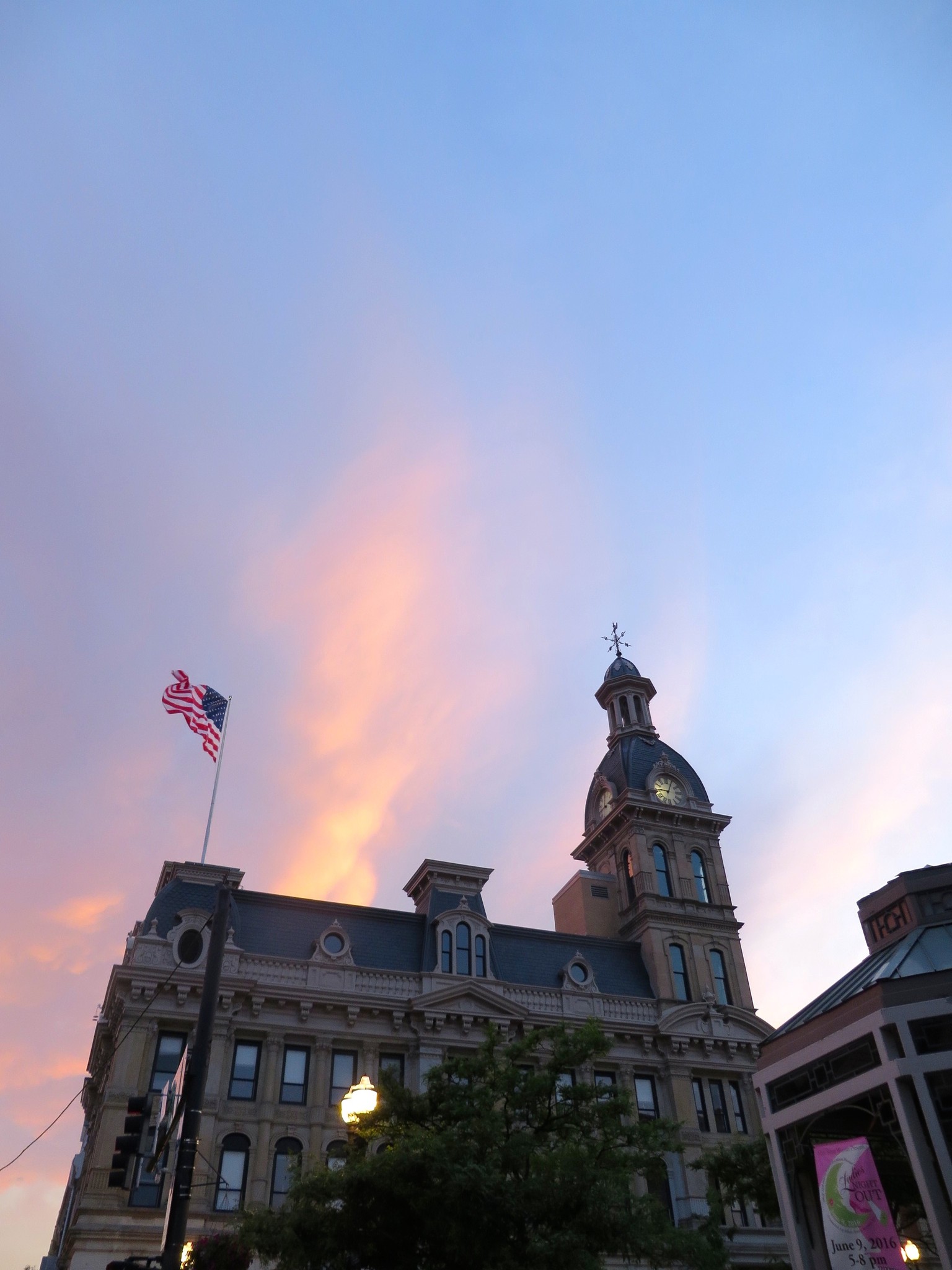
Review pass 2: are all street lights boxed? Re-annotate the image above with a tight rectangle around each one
[338,1076,383,1169]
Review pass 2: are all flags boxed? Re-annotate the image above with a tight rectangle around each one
[161,669,228,764]
[813,1136,909,1270]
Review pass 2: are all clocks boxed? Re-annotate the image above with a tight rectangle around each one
[653,774,686,808]
[598,790,614,821]
